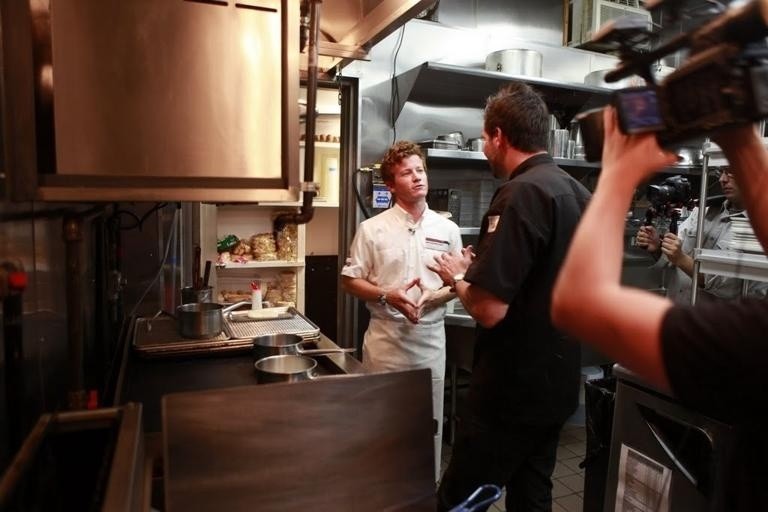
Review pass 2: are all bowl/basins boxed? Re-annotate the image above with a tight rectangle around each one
[438,130,464,148]
[467,137,489,152]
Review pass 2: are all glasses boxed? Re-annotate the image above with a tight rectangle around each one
[715,167,736,178]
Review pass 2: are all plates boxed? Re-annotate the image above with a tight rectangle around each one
[726,217,767,255]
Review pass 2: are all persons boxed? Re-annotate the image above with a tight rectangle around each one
[340,142,464,491]
[550,103,768,512]
[426,80,593,512]
[635,163,768,303]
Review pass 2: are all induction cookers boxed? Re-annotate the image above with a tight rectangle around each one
[128,347,356,450]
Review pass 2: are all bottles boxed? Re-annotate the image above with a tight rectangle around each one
[250,287,265,315]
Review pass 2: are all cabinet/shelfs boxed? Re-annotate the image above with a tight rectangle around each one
[359,61,733,237]
[258,105,342,208]
[219,200,306,314]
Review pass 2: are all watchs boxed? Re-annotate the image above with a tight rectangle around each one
[449,274,464,293]
[377,291,386,305]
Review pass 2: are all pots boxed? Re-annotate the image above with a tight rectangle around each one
[253,355,319,383]
[485,48,545,78]
[249,332,361,363]
[160,300,224,338]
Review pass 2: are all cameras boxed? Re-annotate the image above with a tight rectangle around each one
[647,175,691,206]
